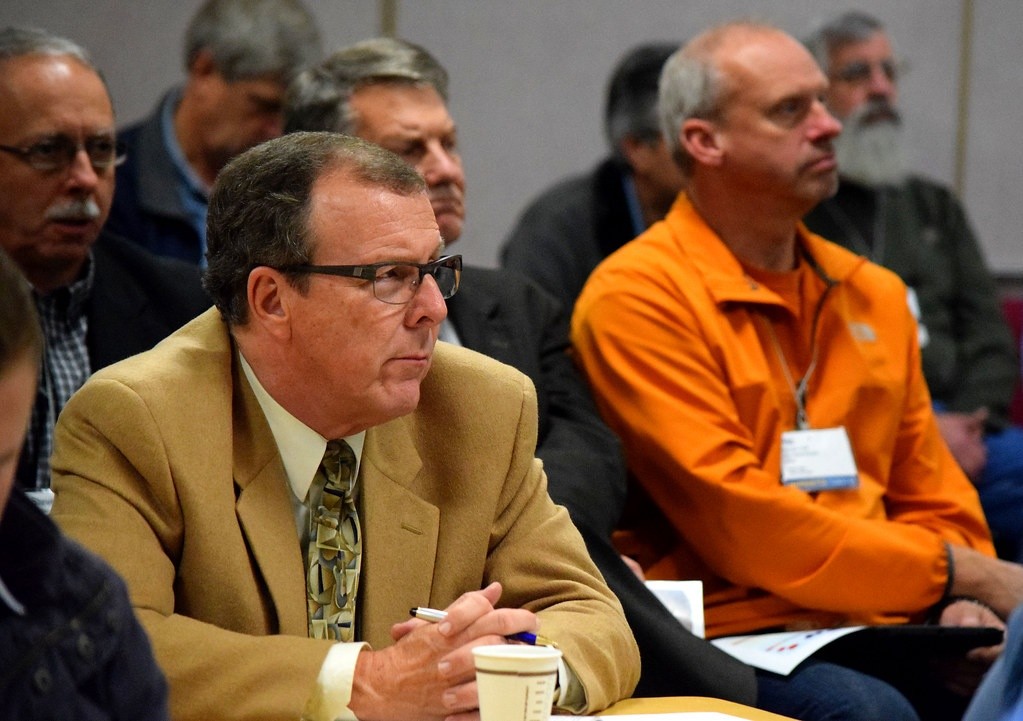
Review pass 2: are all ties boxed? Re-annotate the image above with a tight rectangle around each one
[306,439,365,641]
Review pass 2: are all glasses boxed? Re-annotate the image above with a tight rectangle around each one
[0,137,129,174]
[289,254,462,303]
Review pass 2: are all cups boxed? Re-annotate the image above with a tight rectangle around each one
[471,644,563,721]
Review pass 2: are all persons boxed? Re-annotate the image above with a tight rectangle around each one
[48,130,642,719]
[1,2,1023,546]
[572,23,1023,719]
[0,255,165,721]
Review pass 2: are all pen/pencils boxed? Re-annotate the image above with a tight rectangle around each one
[409,606,557,646]
